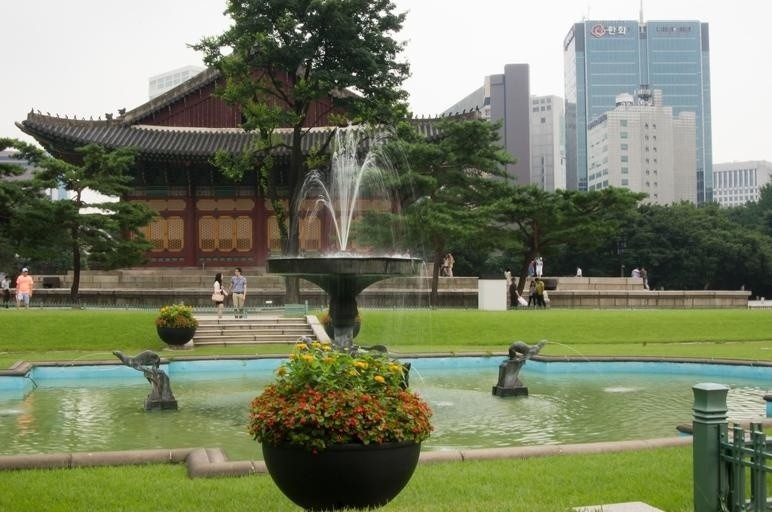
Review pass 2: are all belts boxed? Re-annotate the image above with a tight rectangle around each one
[234,292,244,294]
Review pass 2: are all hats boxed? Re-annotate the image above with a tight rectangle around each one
[22,268,28,272]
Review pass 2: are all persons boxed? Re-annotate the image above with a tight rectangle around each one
[575,264,583,277]
[402,246,410,258]
[631,265,640,278]
[448,253,455,276]
[230,266,249,318]
[212,272,229,319]
[442,255,449,277]
[2,275,12,308]
[504,256,548,309]
[641,266,649,288]
[15,267,34,310]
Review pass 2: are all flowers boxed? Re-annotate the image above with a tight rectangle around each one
[154,303,200,328]
[246,334,436,456]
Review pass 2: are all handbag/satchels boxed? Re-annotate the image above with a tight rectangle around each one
[212,294,224,302]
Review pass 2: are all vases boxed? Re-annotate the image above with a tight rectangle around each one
[261,438,421,511]
[156,327,196,345]
